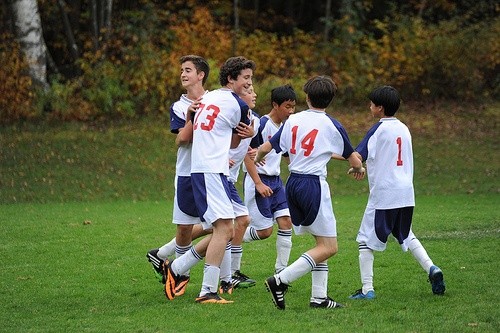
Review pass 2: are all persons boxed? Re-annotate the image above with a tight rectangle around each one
[164,57,256,304]
[243,84,297,284]
[253,75,365,310]
[146,83,261,293]
[346,86,445,299]
[170,54,237,296]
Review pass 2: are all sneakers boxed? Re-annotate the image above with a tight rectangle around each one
[427,265,446,294]
[174,273,190,296]
[232,275,255,288]
[145,248,167,284]
[218,280,233,294]
[349,288,376,298]
[234,270,256,283]
[265,273,290,310]
[310,295,344,308]
[195,292,234,304]
[163,258,179,300]
